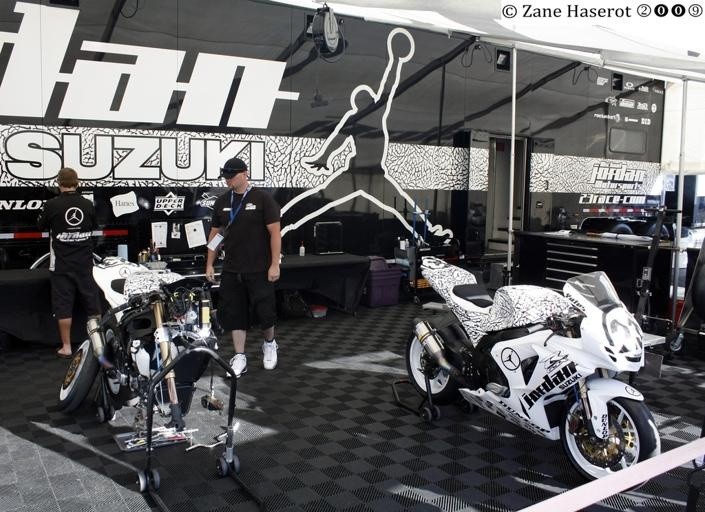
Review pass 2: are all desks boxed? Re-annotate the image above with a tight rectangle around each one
[0,252,371,347]
[628,333,665,388]
[512,229,673,332]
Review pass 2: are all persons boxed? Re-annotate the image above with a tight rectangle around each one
[204,159,283,380]
[35,167,102,359]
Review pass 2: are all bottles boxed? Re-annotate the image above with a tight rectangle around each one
[399,237,409,251]
[300,242,306,257]
[137,242,160,262]
[117,244,128,260]
[555,206,567,228]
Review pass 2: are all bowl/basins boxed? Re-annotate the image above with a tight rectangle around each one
[570,225,581,230]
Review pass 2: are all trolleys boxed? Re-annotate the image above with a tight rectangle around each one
[136,347,270,512]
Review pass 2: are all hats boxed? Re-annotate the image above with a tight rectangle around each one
[217,157,248,181]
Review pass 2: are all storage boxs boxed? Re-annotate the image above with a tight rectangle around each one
[360,256,402,307]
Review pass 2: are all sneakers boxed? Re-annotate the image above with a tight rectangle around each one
[262,338,279,370]
[225,354,249,378]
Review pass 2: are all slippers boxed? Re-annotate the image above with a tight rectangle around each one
[54,346,72,359]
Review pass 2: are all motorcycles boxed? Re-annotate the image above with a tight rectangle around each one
[408,253,662,493]
